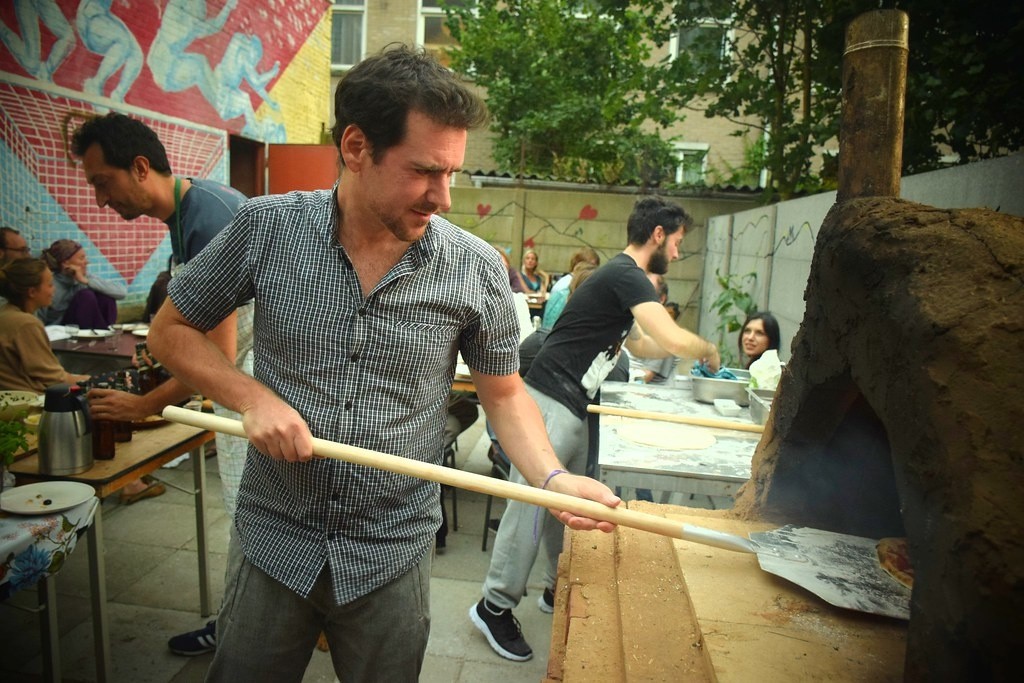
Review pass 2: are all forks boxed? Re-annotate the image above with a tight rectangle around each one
[73,340,97,350]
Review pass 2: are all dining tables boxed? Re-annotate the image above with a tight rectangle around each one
[0,424,40,493]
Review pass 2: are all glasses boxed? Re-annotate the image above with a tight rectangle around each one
[0,246,30,253]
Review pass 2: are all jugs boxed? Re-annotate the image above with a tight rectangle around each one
[39,384,93,476]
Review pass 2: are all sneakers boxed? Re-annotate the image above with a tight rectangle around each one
[168,620,216,655]
[468,596,533,662]
[538,586,554,614]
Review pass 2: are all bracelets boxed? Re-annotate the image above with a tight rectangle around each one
[542,470,569,489]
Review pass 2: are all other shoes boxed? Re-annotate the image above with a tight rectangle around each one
[119,482,166,506]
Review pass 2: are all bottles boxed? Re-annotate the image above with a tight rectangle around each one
[135,343,158,395]
[114,379,132,441]
[143,343,172,386]
[92,383,115,460]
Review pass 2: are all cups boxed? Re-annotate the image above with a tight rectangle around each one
[65,325,79,343]
[105,333,121,351]
[112,324,123,335]
[185,400,204,411]
[151,315,155,323]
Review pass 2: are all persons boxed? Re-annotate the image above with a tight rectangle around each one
[469,198,721,660]
[72,112,256,655]
[0,256,167,503]
[146,42,621,683]
[623,273,680,384]
[0,228,125,330]
[739,313,781,369]
[446,244,631,533]
[143,255,175,323]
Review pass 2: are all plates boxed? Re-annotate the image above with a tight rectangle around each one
[78,330,112,339]
[455,365,471,378]
[0,481,96,515]
[107,324,148,330]
[133,418,166,427]
[132,330,149,336]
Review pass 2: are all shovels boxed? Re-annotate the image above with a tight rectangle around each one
[163,404,913,619]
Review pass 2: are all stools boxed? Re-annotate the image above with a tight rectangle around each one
[481,442,510,551]
[442,446,458,534]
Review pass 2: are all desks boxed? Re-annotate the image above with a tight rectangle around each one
[0,295,765,683]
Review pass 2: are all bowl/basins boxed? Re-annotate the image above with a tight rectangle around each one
[714,398,741,416]
[0,391,38,421]
[24,419,39,430]
